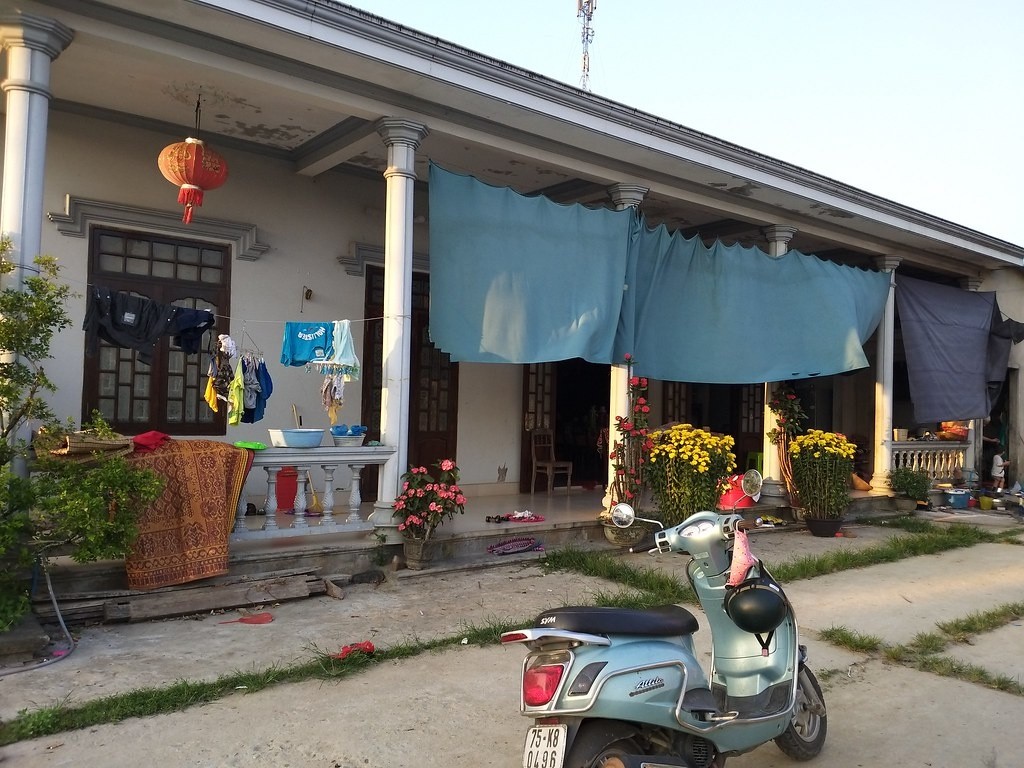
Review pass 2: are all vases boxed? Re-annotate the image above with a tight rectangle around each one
[791,505,808,522]
[802,515,845,537]
[403,531,437,570]
[601,518,647,547]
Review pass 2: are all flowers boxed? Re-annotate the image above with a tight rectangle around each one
[767,384,808,506]
[787,428,857,519]
[392,459,467,540]
[647,421,737,528]
[604,353,655,522]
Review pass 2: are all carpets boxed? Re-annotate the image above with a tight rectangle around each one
[107,439,254,589]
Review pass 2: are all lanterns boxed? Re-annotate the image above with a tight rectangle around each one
[157,95,228,222]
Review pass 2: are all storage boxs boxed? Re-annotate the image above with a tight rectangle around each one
[945,488,970,508]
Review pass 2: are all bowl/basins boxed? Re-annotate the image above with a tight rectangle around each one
[991,498,1009,509]
[968,500,977,507]
[268,428,325,448]
[946,428,970,440]
[979,497,993,509]
[720,475,755,507]
[333,436,365,447]
[993,487,1005,497]
[943,489,969,508]
[893,429,908,441]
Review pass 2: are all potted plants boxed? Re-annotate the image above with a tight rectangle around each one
[883,466,932,511]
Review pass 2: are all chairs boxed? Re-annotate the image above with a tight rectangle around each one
[531,429,573,493]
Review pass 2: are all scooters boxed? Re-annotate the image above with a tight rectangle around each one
[498,468,827,768]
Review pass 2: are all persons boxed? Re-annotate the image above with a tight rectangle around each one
[991,442,1011,493]
[983,416,1000,442]
